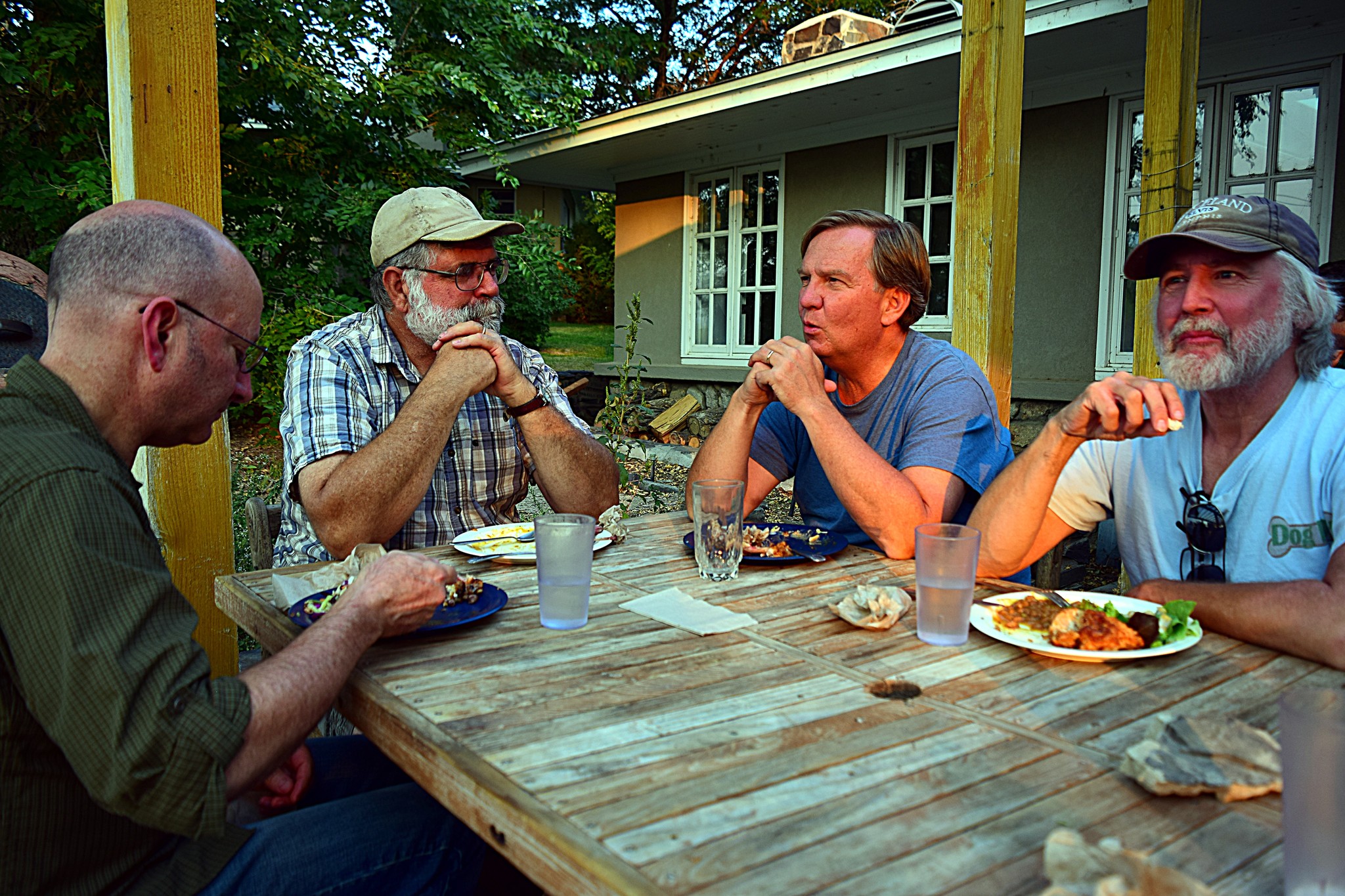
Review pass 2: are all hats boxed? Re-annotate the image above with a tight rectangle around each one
[369,183,526,268]
[1123,193,1323,283]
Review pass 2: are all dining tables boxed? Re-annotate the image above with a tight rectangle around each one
[213,510,1345,896]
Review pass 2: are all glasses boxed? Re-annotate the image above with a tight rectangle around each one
[139,297,269,375]
[399,258,510,292]
[1176,484,1228,585]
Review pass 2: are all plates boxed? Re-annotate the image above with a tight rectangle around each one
[453,522,612,564]
[289,573,508,637]
[969,590,1202,663]
[683,522,848,565]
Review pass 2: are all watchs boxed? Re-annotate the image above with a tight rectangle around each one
[501,385,550,423]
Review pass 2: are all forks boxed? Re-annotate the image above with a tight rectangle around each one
[976,580,1072,609]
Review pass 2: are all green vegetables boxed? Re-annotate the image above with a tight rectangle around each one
[1075,599,1199,648]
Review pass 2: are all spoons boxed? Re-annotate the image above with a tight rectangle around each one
[448,530,536,546]
[766,533,826,562]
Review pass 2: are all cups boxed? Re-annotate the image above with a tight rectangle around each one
[692,478,743,580]
[915,524,981,647]
[1273,682,1344,896]
[533,513,597,630]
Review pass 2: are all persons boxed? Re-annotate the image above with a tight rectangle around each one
[957,195,1343,674]
[685,208,1035,590]
[2,199,541,893]
[275,187,622,736]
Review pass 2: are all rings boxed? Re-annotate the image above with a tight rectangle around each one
[482,327,486,334]
[766,349,773,365]
